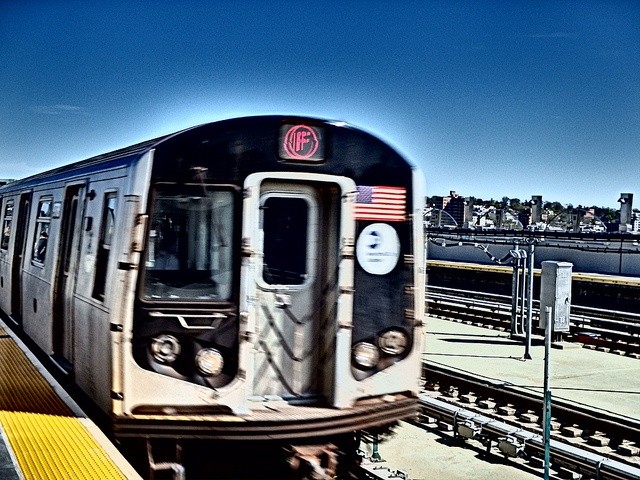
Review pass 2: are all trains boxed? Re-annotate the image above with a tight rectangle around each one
[0,115,425,480]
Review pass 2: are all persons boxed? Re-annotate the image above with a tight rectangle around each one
[4,223,11,249]
[34,239,47,262]
[151,221,179,270]
[41,229,45,232]
[40,232,48,239]
